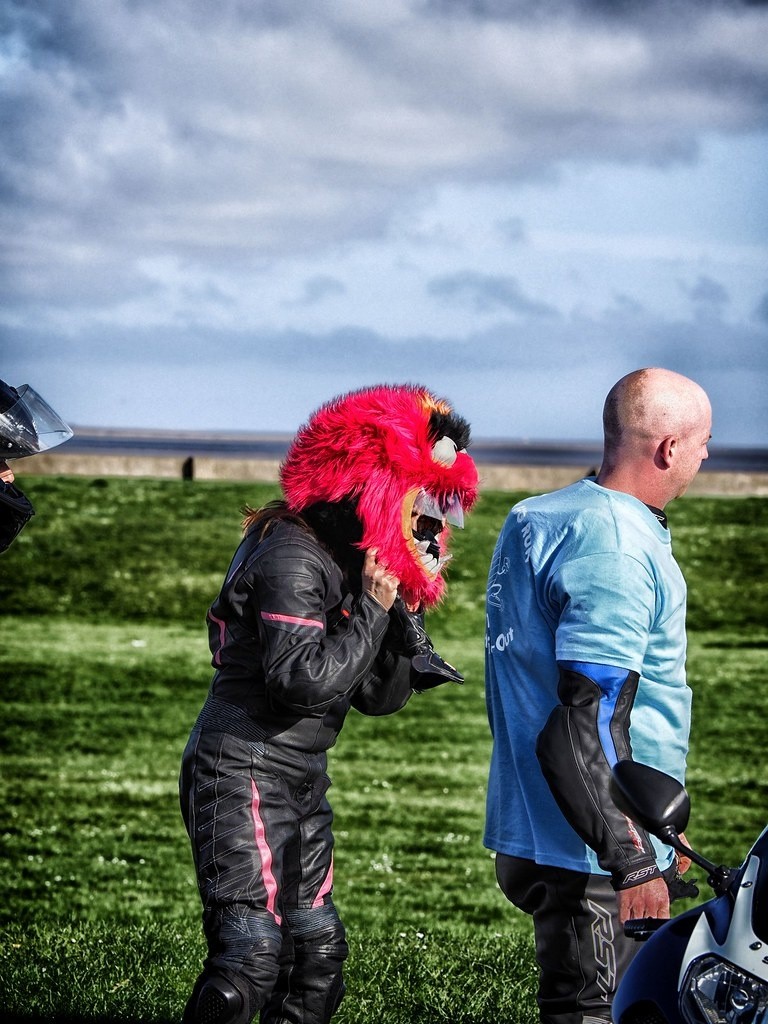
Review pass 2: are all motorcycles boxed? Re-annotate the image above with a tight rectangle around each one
[610,757,768,1024]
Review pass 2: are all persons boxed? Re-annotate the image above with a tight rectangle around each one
[180,384,482,1024]
[0,379,75,555]
[482,369,715,1024]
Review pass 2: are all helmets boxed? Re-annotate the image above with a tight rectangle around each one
[0,378,40,554]
